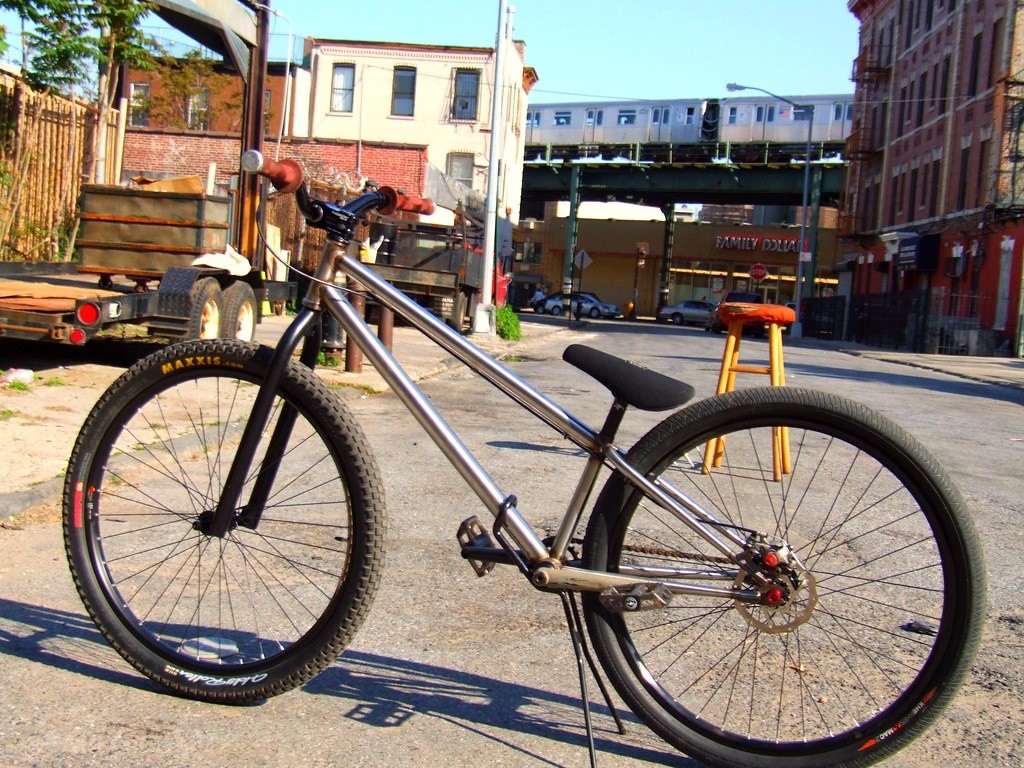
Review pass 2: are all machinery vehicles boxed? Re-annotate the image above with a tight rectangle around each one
[0,177,271,363]
[341,214,510,335]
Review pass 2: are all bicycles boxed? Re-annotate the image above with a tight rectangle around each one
[58,146,987,766]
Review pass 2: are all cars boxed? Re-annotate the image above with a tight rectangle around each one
[713,290,763,333]
[657,299,716,326]
[541,292,622,319]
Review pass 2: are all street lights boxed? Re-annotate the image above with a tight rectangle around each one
[727,82,814,339]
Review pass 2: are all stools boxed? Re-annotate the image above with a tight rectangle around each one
[702,302,797,482]
